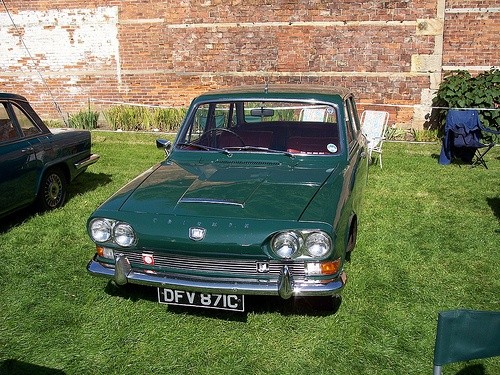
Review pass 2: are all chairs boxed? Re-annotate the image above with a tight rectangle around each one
[0,119,16,142]
[433,309,500,375]
[286,135,340,156]
[219,131,273,149]
[360,110,390,170]
[445,110,500,169]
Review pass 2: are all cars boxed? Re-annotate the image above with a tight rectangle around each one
[88,84,370,315]
[0,93,100,231]
